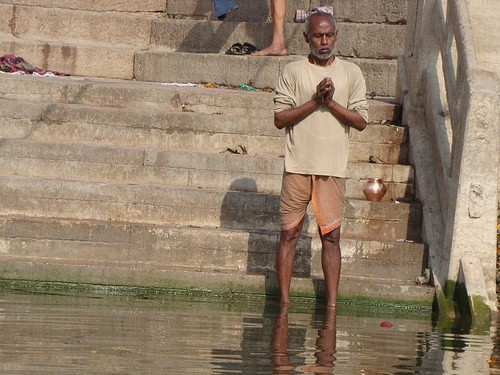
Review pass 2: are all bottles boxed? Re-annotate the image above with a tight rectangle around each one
[363,177,387,203]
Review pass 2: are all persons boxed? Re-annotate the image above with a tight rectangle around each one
[250,0,288,56]
[271,10,370,310]
[269,301,338,375]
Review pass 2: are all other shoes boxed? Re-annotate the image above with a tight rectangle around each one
[226,41,254,54]
